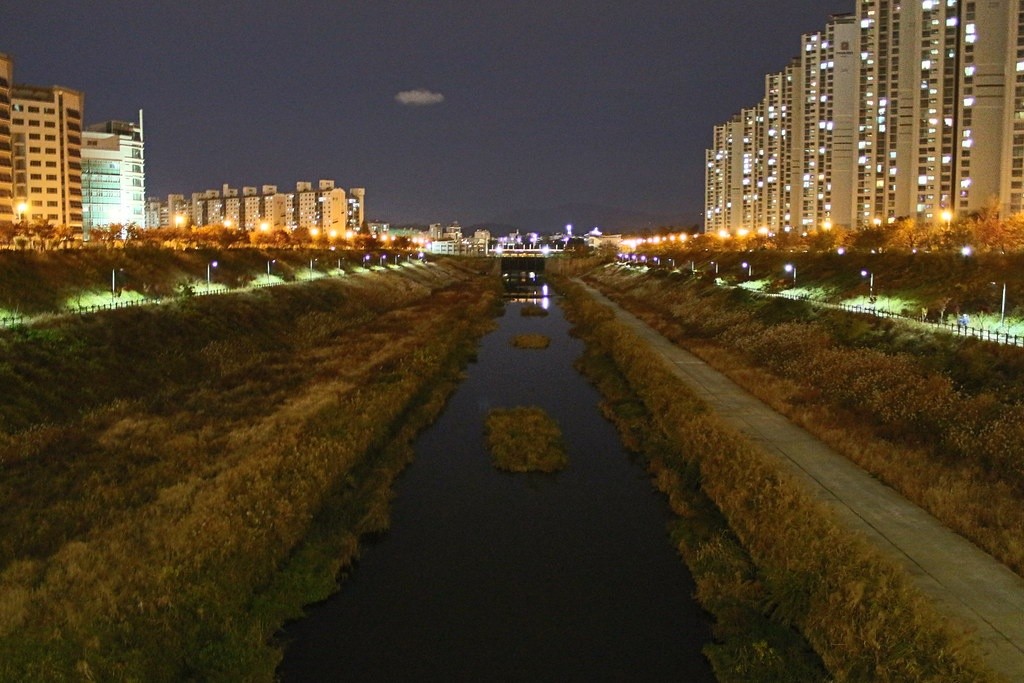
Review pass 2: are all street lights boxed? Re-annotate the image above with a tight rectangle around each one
[785,265,798,287]
[113,266,124,304]
[860,269,874,294]
[310,250,424,279]
[990,281,1006,325]
[616,251,719,276]
[268,259,276,283]
[207,261,219,288]
[741,263,752,278]
